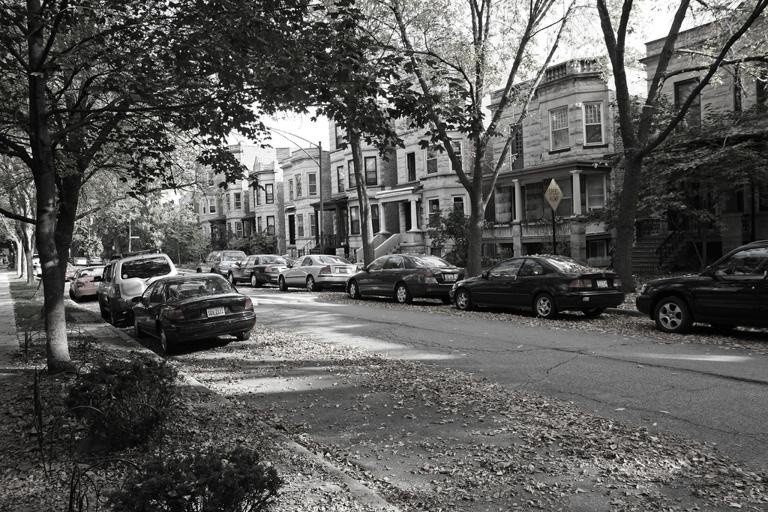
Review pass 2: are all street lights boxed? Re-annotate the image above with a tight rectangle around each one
[267,126,325,255]
